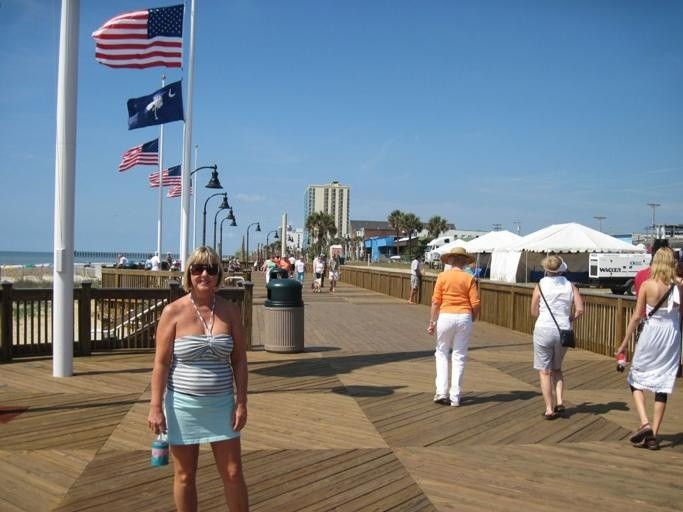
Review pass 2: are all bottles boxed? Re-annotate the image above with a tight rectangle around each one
[150,425,172,468]
[617,351,626,373]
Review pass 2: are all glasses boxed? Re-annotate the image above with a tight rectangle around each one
[188,264,220,278]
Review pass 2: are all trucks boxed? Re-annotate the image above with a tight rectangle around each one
[587,252,651,297]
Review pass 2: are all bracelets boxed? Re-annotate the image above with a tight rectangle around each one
[429,321,436,326]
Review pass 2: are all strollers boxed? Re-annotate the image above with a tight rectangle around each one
[312,272,323,293]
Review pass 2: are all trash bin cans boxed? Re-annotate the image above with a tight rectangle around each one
[263,268,304,353]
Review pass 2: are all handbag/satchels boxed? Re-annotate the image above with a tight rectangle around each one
[635,321,644,341]
[559,329,576,348]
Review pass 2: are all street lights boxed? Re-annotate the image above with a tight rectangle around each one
[593,216,605,232]
[401,229,417,262]
[245,222,259,267]
[212,204,233,253]
[189,163,223,189]
[201,190,229,247]
[266,230,278,260]
[646,203,661,226]
[218,213,236,262]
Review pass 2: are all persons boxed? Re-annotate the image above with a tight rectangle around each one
[147,245,248,512]
[531,255,585,419]
[116,251,174,271]
[408,255,422,305]
[614,246,683,449]
[634,239,670,322]
[427,247,481,408]
[227,253,341,293]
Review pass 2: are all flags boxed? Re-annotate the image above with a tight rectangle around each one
[119,137,159,171]
[148,164,182,187]
[90,4,184,69]
[127,79,184,130]
[168,178,192,198]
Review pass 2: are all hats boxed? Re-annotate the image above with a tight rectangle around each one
[441,247,475,265]
[545,257,569,273]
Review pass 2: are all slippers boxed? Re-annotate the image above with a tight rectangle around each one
[542,412,554,420]
[555,406,565,413]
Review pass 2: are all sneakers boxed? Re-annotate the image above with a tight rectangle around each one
[433,394,446,402]
[634,439,658,450]
[630,424,652,443]
[446,397,460,406]
